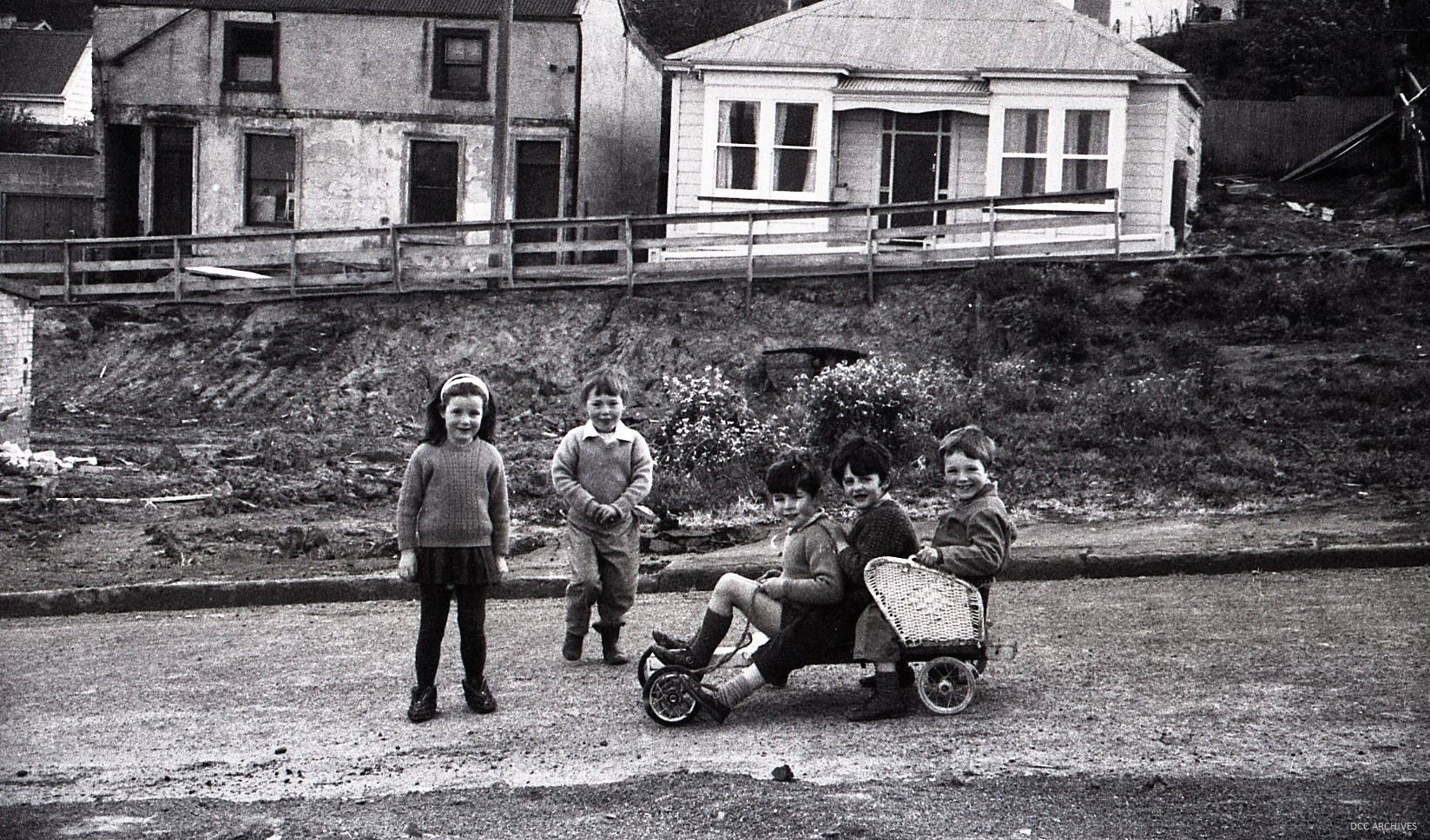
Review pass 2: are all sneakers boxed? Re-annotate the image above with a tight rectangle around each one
[461,675,497,713]
[650,646,705,682]
[407,685,438,721]
[653,628,690,649]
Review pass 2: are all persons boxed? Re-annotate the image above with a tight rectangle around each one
[847,424,1016,723]
[651,455,847,683]
[678,437,920,726]
[395,371,511,722]
[549,366,655,665]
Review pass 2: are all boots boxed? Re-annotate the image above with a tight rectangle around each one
[562,631,583,660]
[593,621,629,664]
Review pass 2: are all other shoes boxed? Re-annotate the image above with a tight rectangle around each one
[859,666,915,688]
[844,692,908,721]
[677,672,732,723]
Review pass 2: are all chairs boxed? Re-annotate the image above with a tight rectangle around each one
[283,171,295,221]
